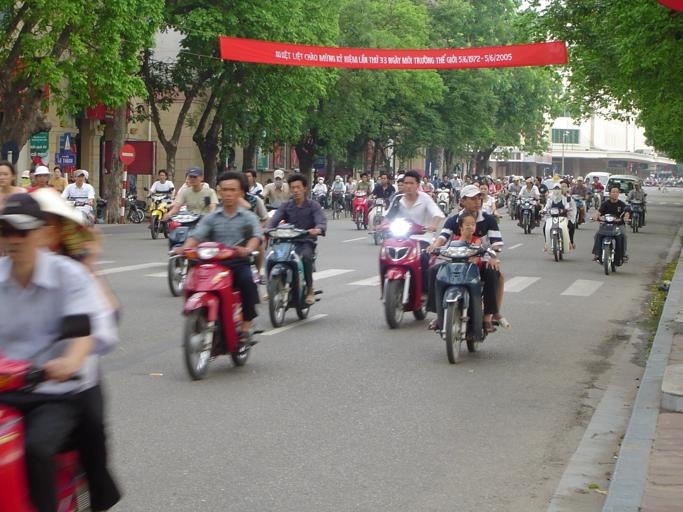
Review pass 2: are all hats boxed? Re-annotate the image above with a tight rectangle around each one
[459,184,484,199]
[0,192,42,233]
[21,170,29,178]
[74,169,89,180]
[187,167,202,178]
[507,173,598,185]
[29,187,83,227]
[33,165,52,176]
[274,168,284,180]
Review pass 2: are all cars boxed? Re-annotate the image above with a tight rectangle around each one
[542,170,642,204]
[498,174,524,184]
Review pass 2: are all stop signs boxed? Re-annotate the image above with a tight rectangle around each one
[119,142,135,167]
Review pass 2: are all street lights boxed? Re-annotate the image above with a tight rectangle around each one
[561,129,569,175]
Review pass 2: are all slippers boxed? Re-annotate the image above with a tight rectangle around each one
[428,317,440,331]
[304,289,315,306]
[482,318,494,334]
[238,326,248,344]
[494,316,512,329]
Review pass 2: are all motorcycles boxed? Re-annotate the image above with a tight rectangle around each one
[0,310,90,510]
[125,193,144,224]
[62,197,89,226]
[94,199,108,224]
